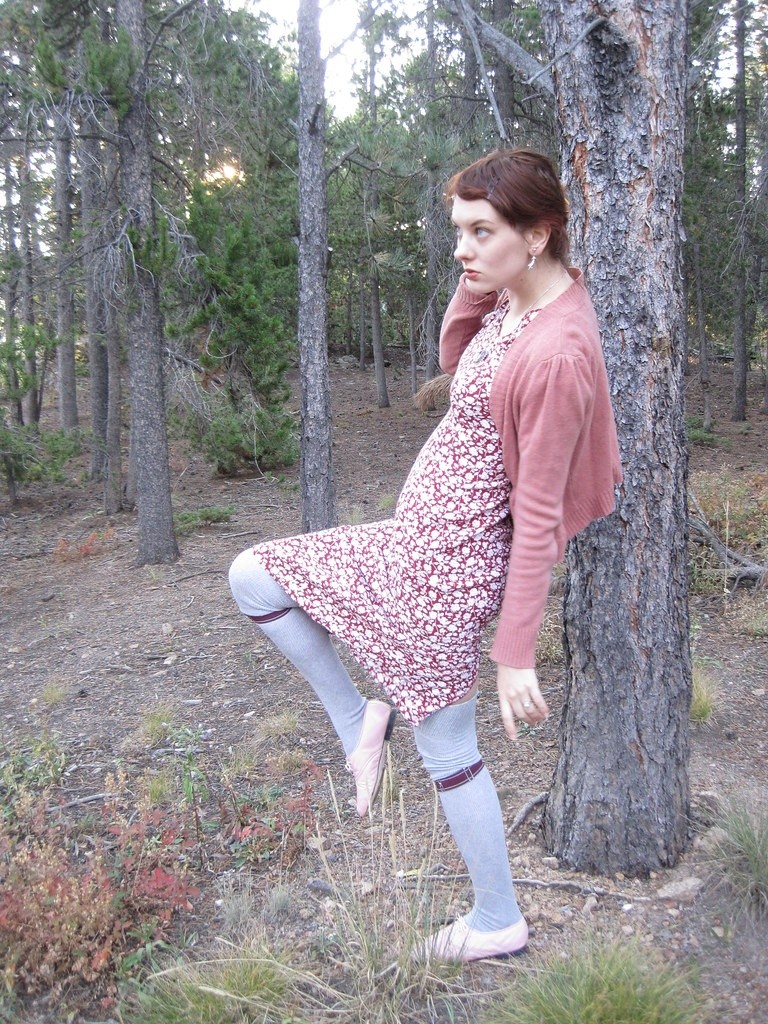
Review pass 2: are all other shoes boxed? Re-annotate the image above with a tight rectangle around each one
[345,698,397,818]
[412,915,529,965]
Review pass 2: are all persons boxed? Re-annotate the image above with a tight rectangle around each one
[229,144,624,963]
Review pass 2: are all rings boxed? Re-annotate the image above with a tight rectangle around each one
[522,700,535,708]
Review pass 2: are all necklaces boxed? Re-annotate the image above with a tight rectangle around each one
[472,272,565,361]
